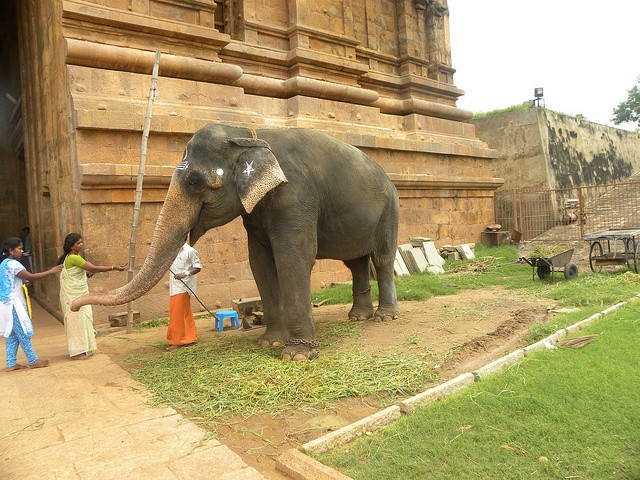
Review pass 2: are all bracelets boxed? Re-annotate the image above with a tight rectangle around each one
[111,266,113,271]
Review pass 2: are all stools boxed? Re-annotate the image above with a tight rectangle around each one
[214,310,240,332]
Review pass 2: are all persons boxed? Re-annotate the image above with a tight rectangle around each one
[165,233,201,351]
[20,227,35,274]
[0,237,62,372]
[56,233,126,360]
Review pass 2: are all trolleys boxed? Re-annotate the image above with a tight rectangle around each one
[585,228,640,273]
[518,249,577,283]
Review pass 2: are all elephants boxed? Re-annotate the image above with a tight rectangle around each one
[71,124,400,362]
